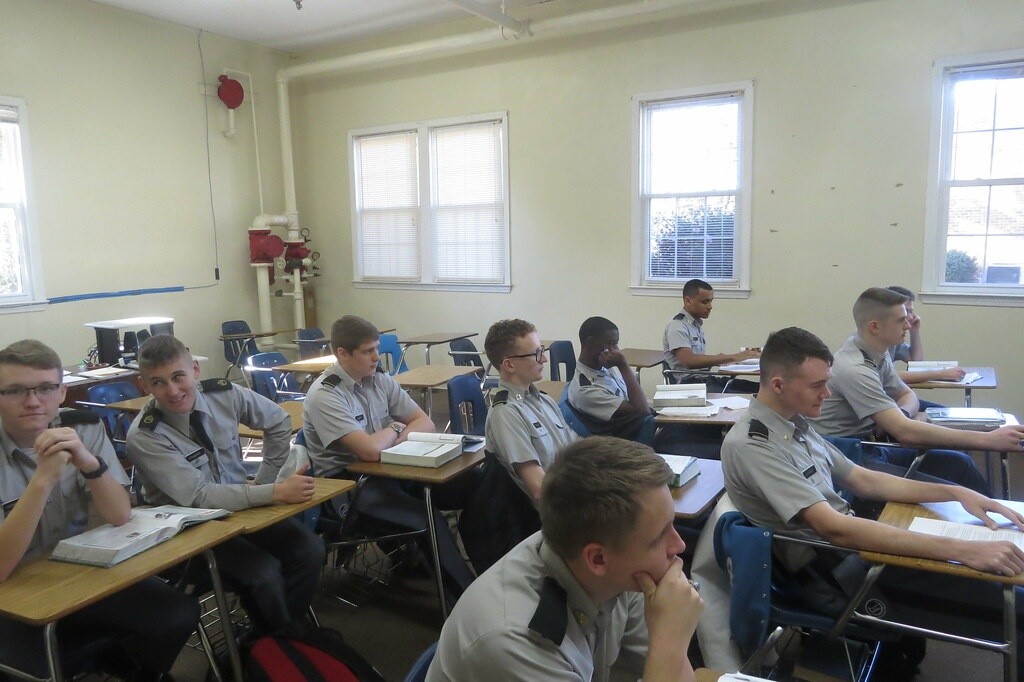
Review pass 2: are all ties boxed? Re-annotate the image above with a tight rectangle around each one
[188,411,214,452]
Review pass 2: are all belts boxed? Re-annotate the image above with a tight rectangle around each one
[857,432,891,443]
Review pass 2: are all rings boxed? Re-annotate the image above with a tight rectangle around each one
[604,348,609,354]
[689,580,701,592]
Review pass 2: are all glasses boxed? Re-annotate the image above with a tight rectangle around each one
[0,380,62,403]
[501,345,545,365]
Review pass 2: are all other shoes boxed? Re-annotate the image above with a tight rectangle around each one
[290,614,317,637]
[377,538,426,581]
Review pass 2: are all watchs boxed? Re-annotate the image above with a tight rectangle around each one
[387,423,403,438]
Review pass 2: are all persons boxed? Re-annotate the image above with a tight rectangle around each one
[0,338,201,682]
[887,284,966,413]
[424,434,705,682]
[663,278,760,394]
[567,316,723,460]
[806,288,1023,617]
[302,315,479,598]
[126,333,326,633]
[484,319,585,532]
[720,326,1024,682]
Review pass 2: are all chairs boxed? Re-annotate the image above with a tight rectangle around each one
[374,333,409,377]
[551,341,577,382]
[714,509,901,682]
[75,380,145,485]
[448,376,490,438]
[298,328,331,362]
[449,339,507,407]
[221,320,261,386]
[242,352,300,462]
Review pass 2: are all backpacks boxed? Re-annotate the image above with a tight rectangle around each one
[242,618,391,681]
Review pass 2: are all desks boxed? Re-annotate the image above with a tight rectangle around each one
[0,328,1023,681]
[58,351,209,425]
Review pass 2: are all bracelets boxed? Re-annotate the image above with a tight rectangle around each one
[79,456,108,480]
